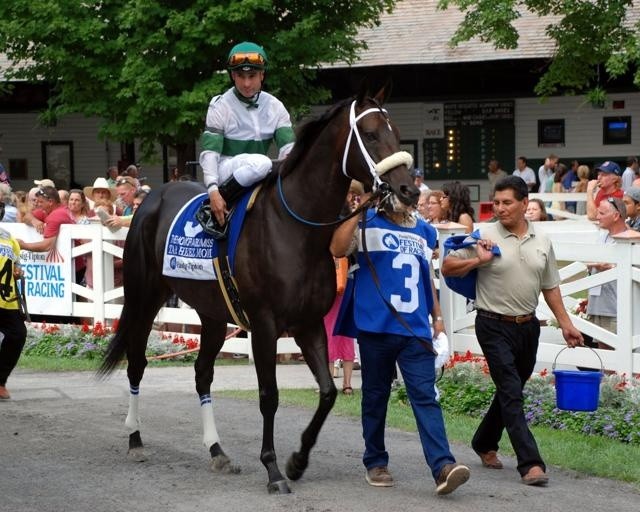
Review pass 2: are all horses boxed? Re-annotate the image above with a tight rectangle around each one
[98,88,420,496]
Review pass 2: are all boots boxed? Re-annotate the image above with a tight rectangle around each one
[195,172,249,241]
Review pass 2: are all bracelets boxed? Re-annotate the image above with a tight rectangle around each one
[433,314,443,325]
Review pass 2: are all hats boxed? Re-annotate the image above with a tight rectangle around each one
[623,186,640,201]
[414,167,423,177]
[594,160,621,176]
[33,179,56,188]
[83,177,119,203]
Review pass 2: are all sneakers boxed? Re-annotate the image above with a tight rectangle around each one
[521,465,549,485]
[470,439,504,470]
[365,466,395,488]
[435,461,470,495]
[0,385,11,401]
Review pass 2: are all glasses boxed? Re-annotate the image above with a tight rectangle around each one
[120,179,136,188]
[442,194,449,198]
[136,188,150,196]
[607,197,622,219]
[228,52,265,66]
[39,189,50,199]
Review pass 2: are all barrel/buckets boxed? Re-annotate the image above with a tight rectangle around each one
[552,342,604,411]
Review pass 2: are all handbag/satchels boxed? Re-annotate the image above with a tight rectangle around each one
[476,310,537,324]
[442,228,501,301]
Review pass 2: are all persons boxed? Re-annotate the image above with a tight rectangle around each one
[1,200,28,400]
[105,165,119,189]
[413,167,429,190]
[511,156,536,193]
[426,190,449,224]
[115,176,136,213]
[441,174,586,486]
[84,177,123,216]
[524,198,547,221]
[417,190,432,225]
[104,190,148,232]
[586,160,624,221]
[622,158,638,192]
[329,205,470,497]
[588,197,637,347]
[14,185,86,281]
[538,155,559,194]
[552,166,571,221]
[68,189,95,216]
[440,180,475,232]
[15,187,38,223]
[621,186,640,233]
[198,40,294,226]
[127,165,141,179]
[561,159,580,214]
[573,165,588,193]
[323,179,365,397]
[486,159,507,201]
[1,183,21,223]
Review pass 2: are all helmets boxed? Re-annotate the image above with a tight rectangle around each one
[226,42,268,73]
